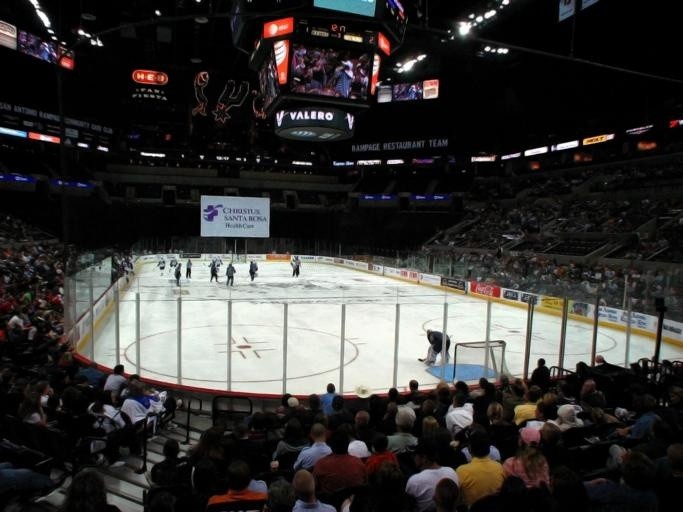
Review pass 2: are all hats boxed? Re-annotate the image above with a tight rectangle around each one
[356,386,371,398]
[557,405,575,418]
[520,427,541,445]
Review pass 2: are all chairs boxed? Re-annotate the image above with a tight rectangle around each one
[144,358,682,512]
[0,352,175,512]
[456,202,656,260]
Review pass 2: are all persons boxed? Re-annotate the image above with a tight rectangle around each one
[2,212,183,512]
[424,329,451,365]
[249,261,256,281]
[292,256,301,277]
[209,256,236,286]
[150,354,681,512]
[421,170,682,320]
[186,259,193,279]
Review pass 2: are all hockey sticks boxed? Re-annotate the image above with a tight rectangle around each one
[418,335,452,362]
